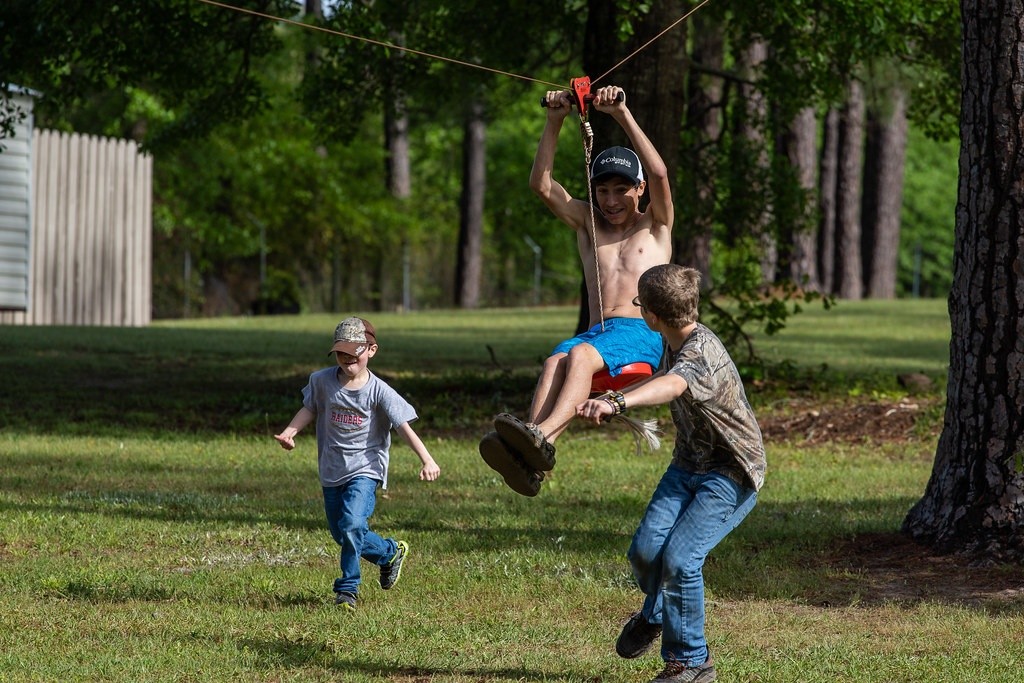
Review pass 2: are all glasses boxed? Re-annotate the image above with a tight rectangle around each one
[631,295,644,308]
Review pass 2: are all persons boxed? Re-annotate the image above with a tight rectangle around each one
[478,85,674,498]
[273,316,440,613]
[573,263,767,683]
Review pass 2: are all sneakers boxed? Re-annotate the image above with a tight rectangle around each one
[647,645,716,683]
[379,540,410,590]
[336,589,359,609]
[615,612,664,659]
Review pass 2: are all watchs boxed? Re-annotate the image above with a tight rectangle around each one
[609,391,627,413]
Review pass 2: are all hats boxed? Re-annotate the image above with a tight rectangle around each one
[327,316,377,359]
[590,145,644,185]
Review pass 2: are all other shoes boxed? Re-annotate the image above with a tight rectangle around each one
[494,413,557,471]
[479,430,546,497]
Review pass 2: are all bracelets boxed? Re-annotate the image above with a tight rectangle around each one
[604,397,621,417]
